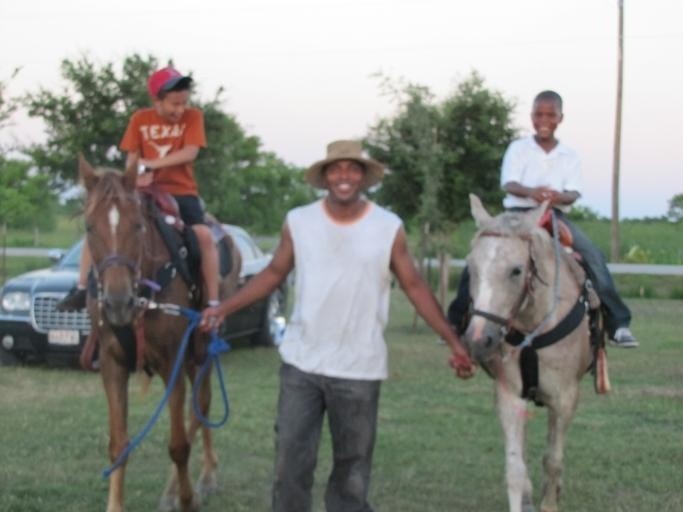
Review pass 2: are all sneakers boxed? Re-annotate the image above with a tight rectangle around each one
[57,284,88,313]
[608,327,639,348]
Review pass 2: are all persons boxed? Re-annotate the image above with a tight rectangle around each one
[53,66,226,334]
[194,139,475,512]
[433,89,639,351]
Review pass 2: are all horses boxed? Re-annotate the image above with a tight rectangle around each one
[458,193,609,512]
[77,149,243,512]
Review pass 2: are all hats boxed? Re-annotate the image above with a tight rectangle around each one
[147,67,192,100]
[304,140,385,190]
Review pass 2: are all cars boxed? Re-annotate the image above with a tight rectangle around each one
[0,221,288,365]
